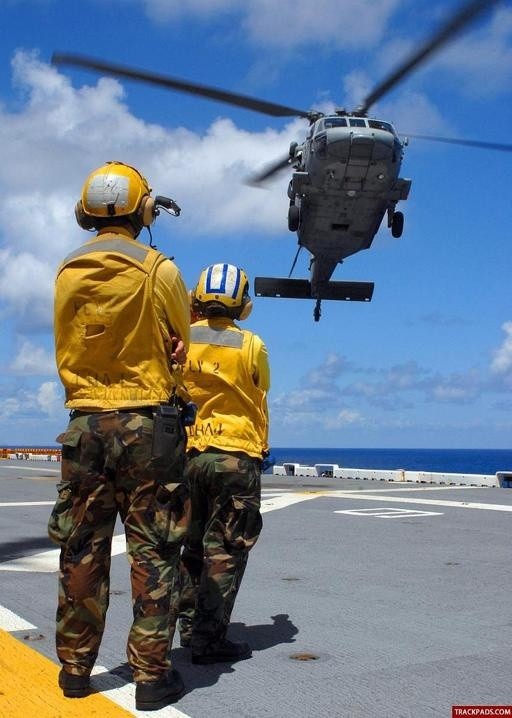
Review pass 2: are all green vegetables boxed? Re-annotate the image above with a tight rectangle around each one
[50,0,511,322]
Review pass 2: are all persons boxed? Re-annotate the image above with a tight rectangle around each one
[47,161,198,711]
[174,262,268,665]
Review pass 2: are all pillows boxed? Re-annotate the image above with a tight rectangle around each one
[82,163,151,238]
[196,263,249,310]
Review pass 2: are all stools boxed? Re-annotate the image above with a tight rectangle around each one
[69,408,157,416]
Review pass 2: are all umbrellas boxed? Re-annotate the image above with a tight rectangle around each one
[191,638,252,665]
[136,670,184,711]
[179,639,190,647]
[59,666,89,696]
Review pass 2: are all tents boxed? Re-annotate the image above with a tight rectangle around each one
[233,294,253,321]
[187,289,199,316]
[75,161,160,230]
